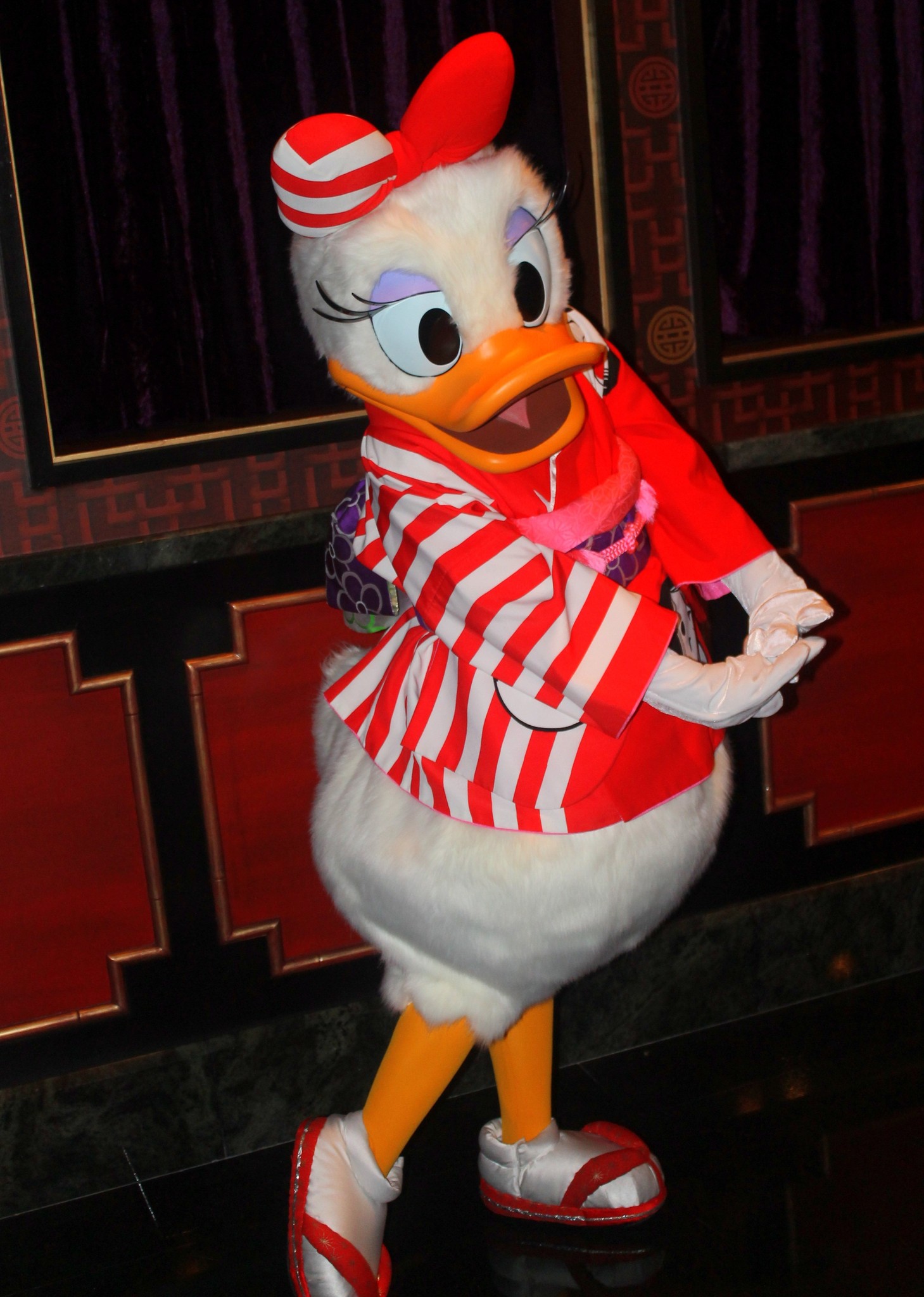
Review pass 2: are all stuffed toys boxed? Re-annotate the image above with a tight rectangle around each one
[266,31,834,1297]
